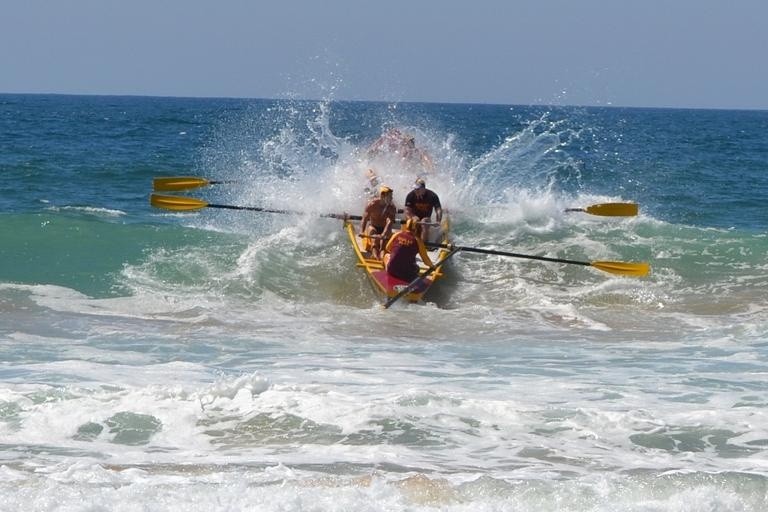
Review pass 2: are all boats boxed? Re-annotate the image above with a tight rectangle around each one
[343,125,457,308]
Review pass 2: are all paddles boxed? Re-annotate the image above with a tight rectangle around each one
[397,204,640,216]
[151,194,440,228]
[359,235,649,278]
[153,176,244,191]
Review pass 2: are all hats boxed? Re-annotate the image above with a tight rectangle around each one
[412,179,424,190]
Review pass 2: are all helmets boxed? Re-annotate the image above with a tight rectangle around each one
[406,218,416,230]
[379,186,392,194]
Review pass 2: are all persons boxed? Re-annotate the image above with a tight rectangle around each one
[358,185,397,260]
[402,179,443,248]
[382,217,437,278]
